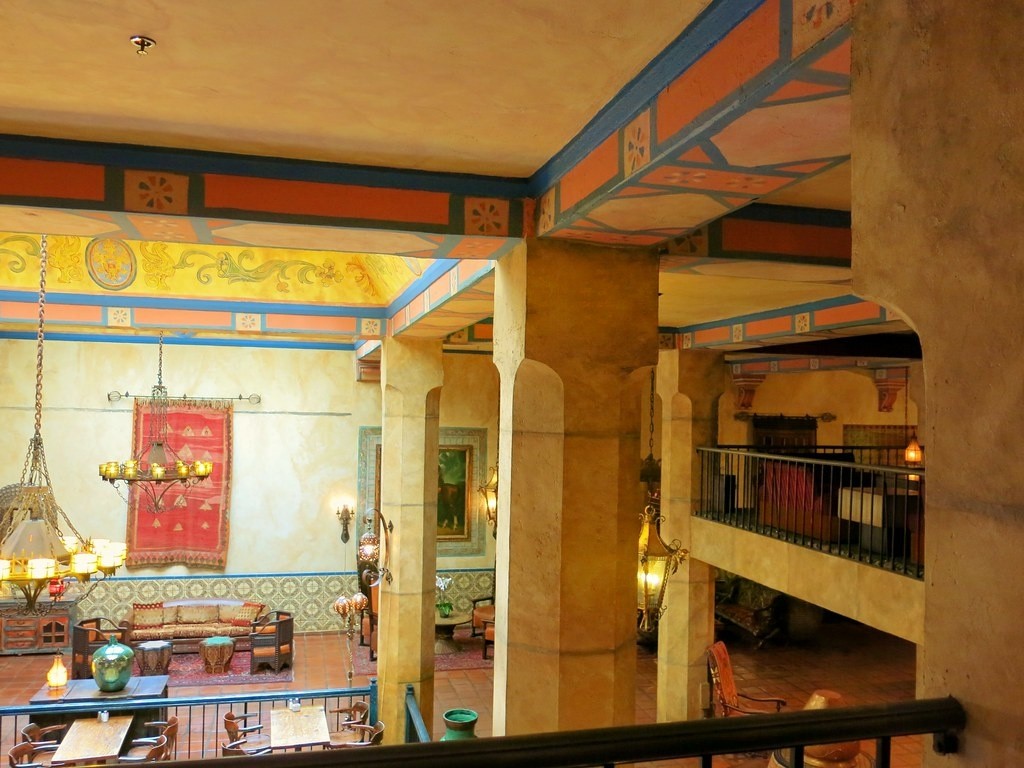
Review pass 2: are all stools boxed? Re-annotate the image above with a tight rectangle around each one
[471,595,495,659]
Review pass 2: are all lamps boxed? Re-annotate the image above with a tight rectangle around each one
[476,462,498,538]
[0,231,127,616]
[636,366,688,630]
[47,647,68,690]
[336,501,396,588]
[98,331,213,513]
[905,429,921,461]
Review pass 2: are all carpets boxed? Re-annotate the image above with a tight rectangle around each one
[61,651,295,687]
[351,627,494,675]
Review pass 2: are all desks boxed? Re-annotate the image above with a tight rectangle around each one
[839,487,918,556]
[50,715,133,767]
[199,634,236,674]
[29,673,169,757]
[133,640,173,675]
[270,705,330,751]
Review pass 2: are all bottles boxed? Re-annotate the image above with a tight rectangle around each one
[91,634,134,692]
[288,698,300,712]
[97,710,110,722]
[439,708,481,741]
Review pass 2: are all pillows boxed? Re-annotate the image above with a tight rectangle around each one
[133,602,164,630]
[231,602,266,625]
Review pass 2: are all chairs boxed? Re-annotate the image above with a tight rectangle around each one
[221,701,385,755]
[702,641,786,768]
[249,611,295,673]
[72,618,130,679]
[7,716,179,768]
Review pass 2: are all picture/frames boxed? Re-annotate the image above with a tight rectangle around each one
[374,444,473,544]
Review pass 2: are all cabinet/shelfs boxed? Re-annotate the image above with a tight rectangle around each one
[0,601,77,655]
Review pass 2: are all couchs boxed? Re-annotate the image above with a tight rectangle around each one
[754,452,923,567]
[119,599,270,653]
